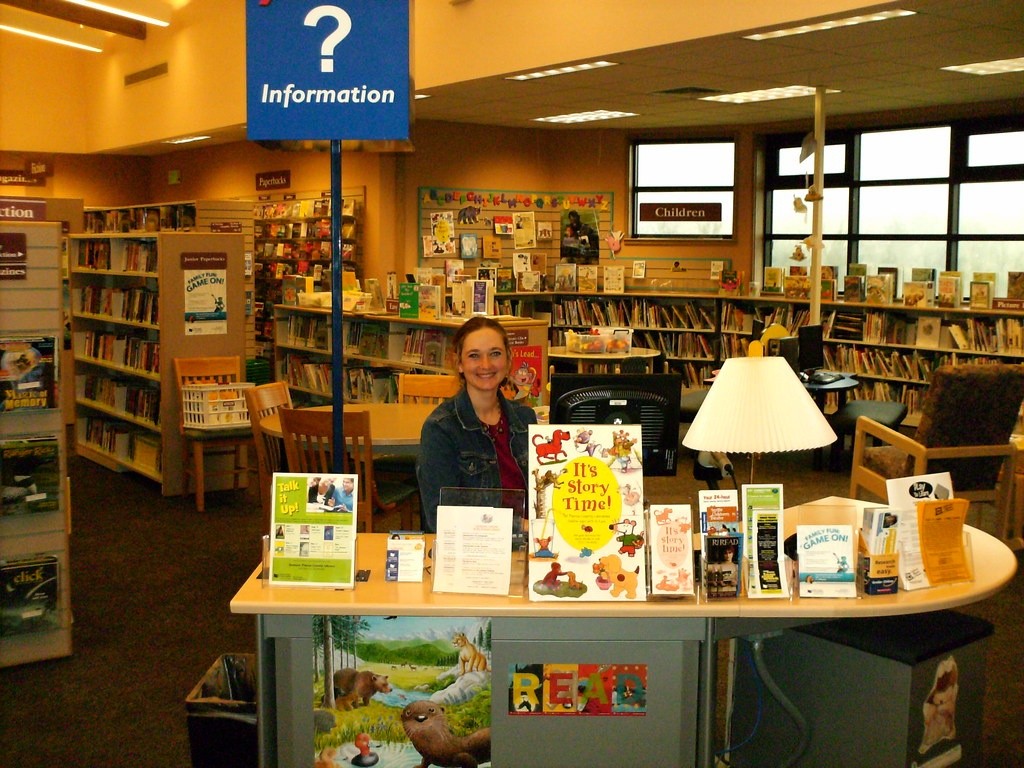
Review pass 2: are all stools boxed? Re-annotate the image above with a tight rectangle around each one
[839,400,908,472]
[679,387,711,423]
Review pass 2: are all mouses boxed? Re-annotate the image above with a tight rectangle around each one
[834,374,845,379]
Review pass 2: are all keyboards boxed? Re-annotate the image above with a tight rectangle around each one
[810,372,837,383]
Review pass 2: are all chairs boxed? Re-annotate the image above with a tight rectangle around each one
[849,363,1024,560]
[173,356,462,540]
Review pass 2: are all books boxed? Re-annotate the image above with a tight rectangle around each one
[477,253,716,390]
[0,332,62,636]
[60,202,196,470]
[720,264,1023,416]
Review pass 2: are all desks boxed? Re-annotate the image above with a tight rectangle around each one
[549,346,662,375]
[227,498,1017,768]
[801,374,858,470]
[259,403,443,536]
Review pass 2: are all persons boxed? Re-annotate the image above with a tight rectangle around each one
[309,477,353,512]
[280,274,493,400]
[419,318,538,531]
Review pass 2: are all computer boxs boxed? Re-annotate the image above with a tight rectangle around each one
[768,336,800,379]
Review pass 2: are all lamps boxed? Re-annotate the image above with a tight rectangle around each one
[682,357,838,486]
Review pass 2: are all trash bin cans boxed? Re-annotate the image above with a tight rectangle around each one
[246,358,272,387]
[184,652,259,768]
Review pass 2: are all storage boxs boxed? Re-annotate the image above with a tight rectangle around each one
[593,325,634,352]
[180,381,254,433]
[563,331,606,354]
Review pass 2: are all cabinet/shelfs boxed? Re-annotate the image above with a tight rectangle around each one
[269,293,1024,424]
[2,197,253,672]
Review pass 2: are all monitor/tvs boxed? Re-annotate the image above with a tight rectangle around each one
[549,373,681,477]
[799,324,825,377]
